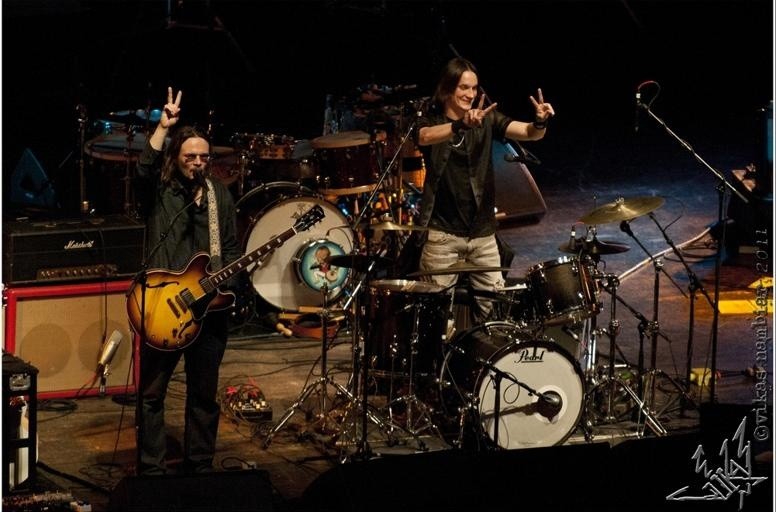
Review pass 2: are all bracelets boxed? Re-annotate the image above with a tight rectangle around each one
[533,122,546,130]
[451,118,473,138]
[534,118,548,125]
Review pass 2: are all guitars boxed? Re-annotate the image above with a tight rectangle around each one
[126,203,325,351]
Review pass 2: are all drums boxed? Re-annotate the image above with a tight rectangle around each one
[252,140,319,194]
[211,145,254,203]
[235,181,361,313]
[312,130,392,196]
[364,279,455,397]
[439,321,587,450]
[291,238,351,295]
[528,255,604,327]
[83,130,172,217]
[392,141,427,188]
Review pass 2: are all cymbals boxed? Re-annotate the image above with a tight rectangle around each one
[557,236,632,254]
[407,259,515,276]
[324,254,396,271]
[350,222,437,231]
[576,195,666,224]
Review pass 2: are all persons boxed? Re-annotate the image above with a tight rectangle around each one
[309,246,331,284]
[410,54,555,404]
[129,84,241,487]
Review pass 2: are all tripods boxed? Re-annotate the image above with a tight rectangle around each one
[300,323,432,452]
[253,312,384,448]
[634,313,698,427]
[379,318,445,441]
[585,335,664,437]
[656,293,720,421]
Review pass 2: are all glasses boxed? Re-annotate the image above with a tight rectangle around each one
[182,153,211,166]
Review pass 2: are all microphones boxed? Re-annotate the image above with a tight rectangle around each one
[192,168,213,191]
[416,93,431,120]
[632,93,643,135]
[97,330,125,377]
[525,383,564,420]
